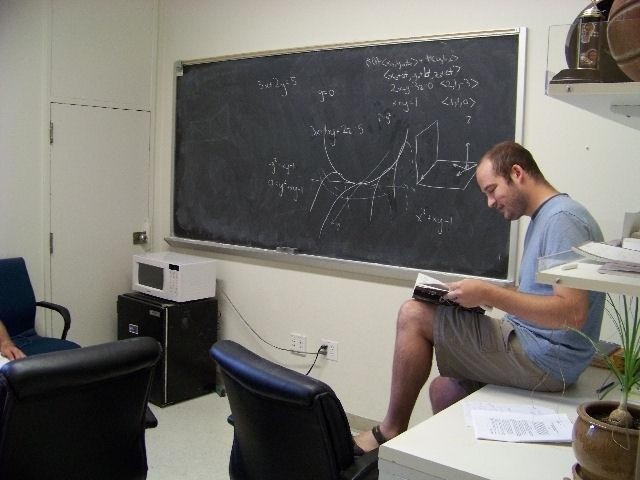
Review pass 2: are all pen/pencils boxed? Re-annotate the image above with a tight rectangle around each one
[596,381,614,393]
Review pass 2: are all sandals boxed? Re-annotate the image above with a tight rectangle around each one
[351,425,388,457]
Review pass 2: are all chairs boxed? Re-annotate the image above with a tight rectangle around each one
[1,257,81,358]
[0,337,158,480]
[208,340,378,480]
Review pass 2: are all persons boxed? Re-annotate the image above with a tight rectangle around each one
[350,140,606,459]
[0,320,28,370]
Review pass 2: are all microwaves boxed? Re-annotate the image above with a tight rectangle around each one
[131,251,216,303]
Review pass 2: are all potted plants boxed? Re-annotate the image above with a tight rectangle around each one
[556,292,639,480]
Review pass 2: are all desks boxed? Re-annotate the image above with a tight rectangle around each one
[378,366,640,480]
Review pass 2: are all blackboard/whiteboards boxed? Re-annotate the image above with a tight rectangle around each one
[163,25,527,290]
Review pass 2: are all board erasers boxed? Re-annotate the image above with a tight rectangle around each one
[275,246,299,255]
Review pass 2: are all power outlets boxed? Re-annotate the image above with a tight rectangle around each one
[289,333,306,356]
[316,338,338,361]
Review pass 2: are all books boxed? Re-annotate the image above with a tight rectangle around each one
[595,339,640,362]
[410,272,489,316]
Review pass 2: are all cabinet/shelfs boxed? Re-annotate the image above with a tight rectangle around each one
[534,21,640,297]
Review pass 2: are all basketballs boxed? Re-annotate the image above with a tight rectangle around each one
[608,1,640,82]
